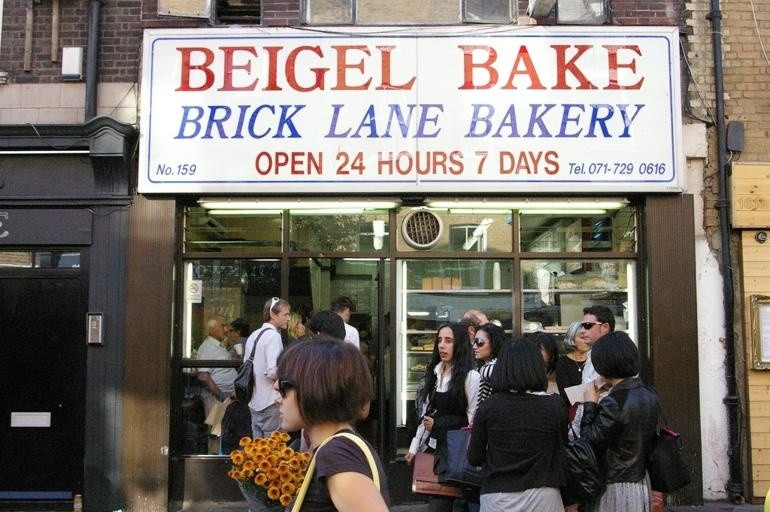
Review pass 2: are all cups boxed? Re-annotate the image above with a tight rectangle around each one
[233,343,243,356]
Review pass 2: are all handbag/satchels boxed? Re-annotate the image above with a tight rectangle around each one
[445,429,481,491]
[646,428,690,493]
[234,361,252,404]
[412,452,462,498]
[561,438,606,507]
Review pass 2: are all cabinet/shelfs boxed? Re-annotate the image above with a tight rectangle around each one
[524,288,630,336]
[406,288,513,393]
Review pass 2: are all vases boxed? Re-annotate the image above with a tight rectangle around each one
[234,471,286,512]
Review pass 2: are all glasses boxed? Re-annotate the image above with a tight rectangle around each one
[279,381,292,398]
[580,322,603,330]
[269,297,280,313]
[474,338,485,348]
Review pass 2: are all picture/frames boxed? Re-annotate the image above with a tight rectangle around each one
[750,295,770,371]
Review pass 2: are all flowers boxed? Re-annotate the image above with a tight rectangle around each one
[227,430,312,507]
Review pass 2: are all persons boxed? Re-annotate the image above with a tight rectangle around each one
[198,298,396,512]
[409,304,664,512]
